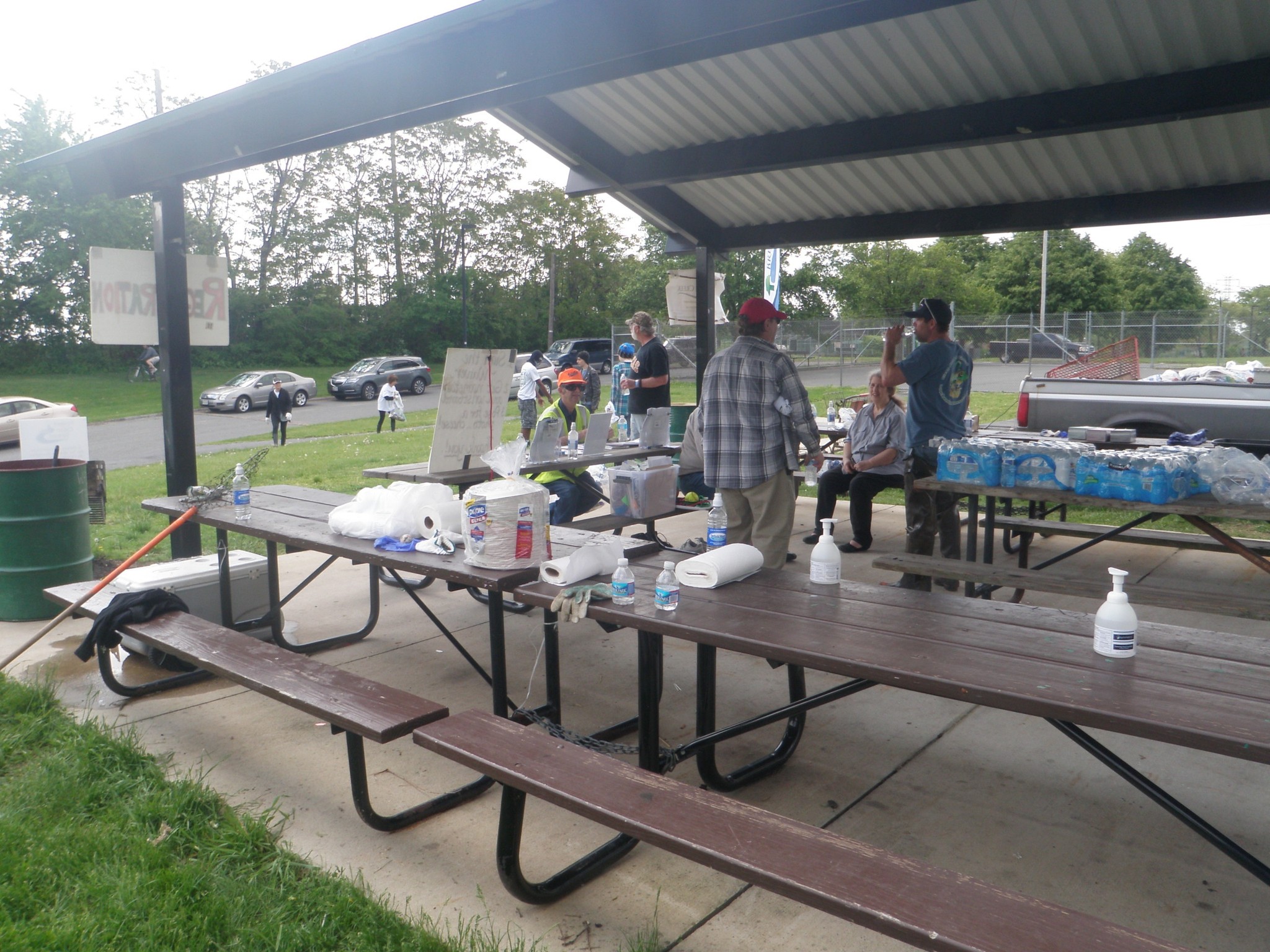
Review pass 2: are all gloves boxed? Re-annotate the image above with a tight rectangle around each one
[550,589,592,623]
[265,417,269,424]
[395,396,399,399]
[562,582,613,604]
[286,412,292,420]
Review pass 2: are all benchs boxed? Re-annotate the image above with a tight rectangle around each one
[874,553,1270,632]
[979,515,1270,603]
[465,509,699,614]
[414,708,1192,952]
[796,453,843,472]
[47,578,450,831]
[793,472,906,504]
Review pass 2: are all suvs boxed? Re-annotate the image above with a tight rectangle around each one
[663,336,697,367]
[327,356,431,400]
[508,353,558,398]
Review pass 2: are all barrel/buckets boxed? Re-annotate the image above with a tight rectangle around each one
[0,458,93,622]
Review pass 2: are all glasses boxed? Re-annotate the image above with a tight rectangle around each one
[770,318,780,324]
[560,383,586,391]
[629,324,636,329]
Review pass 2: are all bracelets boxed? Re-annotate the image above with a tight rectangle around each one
[639,378,643,389]
[635,379,639,388]
[548,397,553,401]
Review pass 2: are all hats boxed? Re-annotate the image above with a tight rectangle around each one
[557,368,588,388]
[561,362,572,371]
[625,312,653,328]
[904,298,953,324]
[530,350,543,365]
[577,350,590,364]
[739,297,787,324]
[273,377,282,384]
[617,342,634,358]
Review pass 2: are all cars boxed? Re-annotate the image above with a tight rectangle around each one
[200,370,316,414]
[0,395,79,446]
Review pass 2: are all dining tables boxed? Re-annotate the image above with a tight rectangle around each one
[138,480,666,782]
[987,431,1270,541]
[510,549,1270,952]
[361,441,685,597]
[816,423,998,441]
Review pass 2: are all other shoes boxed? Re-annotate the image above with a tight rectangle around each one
[838,542,868,553]
[803,535,819,545]
[880,580,901,588]
[932,576,958,592]
[786,553,797,562]
[273,445,278,447]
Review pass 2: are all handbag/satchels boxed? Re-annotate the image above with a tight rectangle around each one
[388,391,406,422]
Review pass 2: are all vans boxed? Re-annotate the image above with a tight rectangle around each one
[544,337,618,375]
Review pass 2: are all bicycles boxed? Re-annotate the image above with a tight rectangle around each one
[128,358,160,383]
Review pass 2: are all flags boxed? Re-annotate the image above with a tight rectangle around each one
[762,249,780,311]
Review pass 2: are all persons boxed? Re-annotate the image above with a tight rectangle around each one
[535,367,616,525]
[516,350,555,446]
[574,349,602,413]
[605,343,638,436]
[620,310,672,441]
[801,368,914,552]
[876,296,975,592]
[677,404,798,562]
[698,299,825,569]
[264,377,292,448]
[135,344,161,382]
[374,373,399,434]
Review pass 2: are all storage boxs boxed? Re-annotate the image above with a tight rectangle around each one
[109,549,286,668]
[605,463,682,518]
[1069,427,1136,445]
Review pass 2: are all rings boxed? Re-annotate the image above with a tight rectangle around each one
[623,386,627,389]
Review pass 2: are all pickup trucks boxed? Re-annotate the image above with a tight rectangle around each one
[1015,377,1270,451]
[990,333,1096,363]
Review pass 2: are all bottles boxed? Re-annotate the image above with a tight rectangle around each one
[612,558,636,604]
[811,404,817,422]
[620,373,630,395]
[882,325,916,342]
[233,463,252,520]
[827,401,836,430]
[805,459,818,486]
[1093,567,1138,657]
[618,415,628,442]
[567,422,579,459]
[810,518,842,584]
[937,436,1217,504]
[706,492,726,551]
[656,561,680,610]
